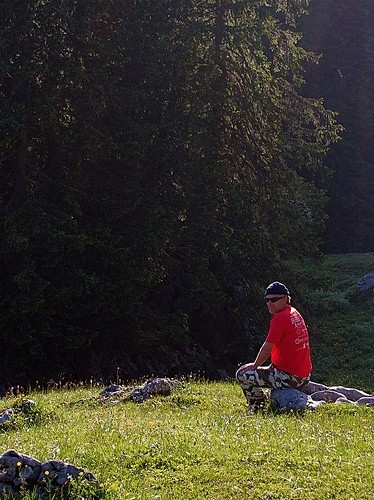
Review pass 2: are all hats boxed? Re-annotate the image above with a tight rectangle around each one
[265,282,289,296]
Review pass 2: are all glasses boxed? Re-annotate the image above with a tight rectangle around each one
[264,295,286,302]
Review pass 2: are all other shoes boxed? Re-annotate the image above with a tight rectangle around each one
[245,398,266,413]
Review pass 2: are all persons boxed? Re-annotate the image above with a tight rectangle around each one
[235,280,313,414]
